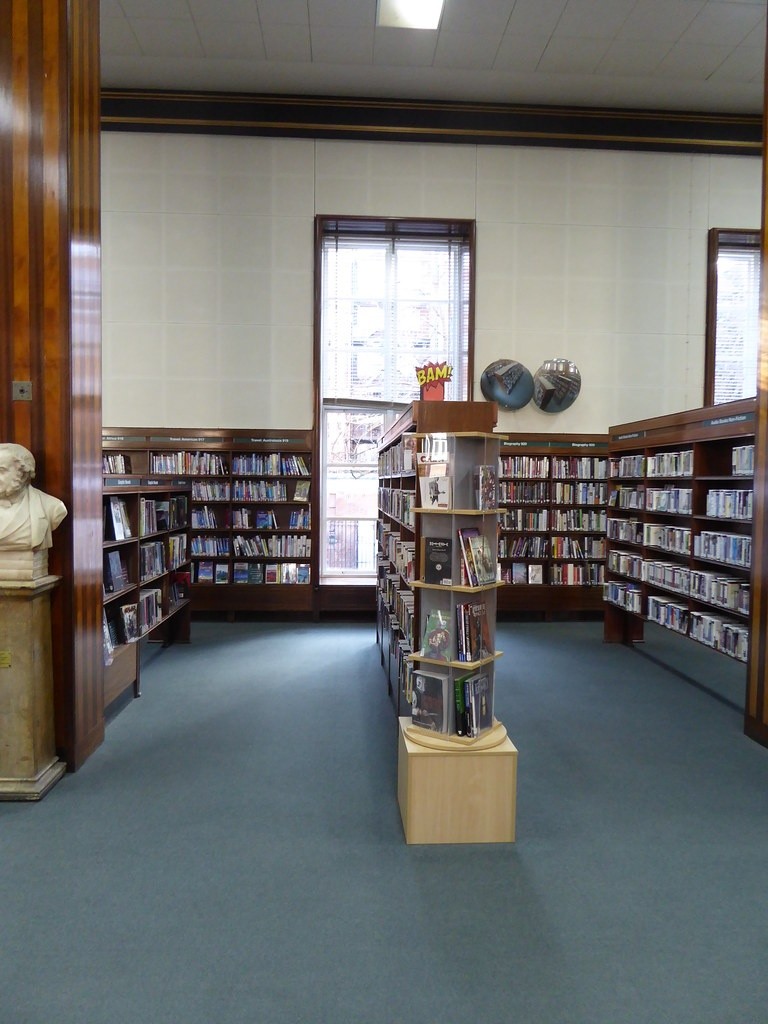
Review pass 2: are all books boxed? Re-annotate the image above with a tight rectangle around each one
[484,361,523,396]
[602,443,755,664]
[327,518,376,570]
[454,671,493,740]
[474,463,499,511]
[377,431,454,736]
[456,525,495,587]
[532,370,581,409]
[102,450,313,668]
[455,599,494,663]
[495,456,606,587]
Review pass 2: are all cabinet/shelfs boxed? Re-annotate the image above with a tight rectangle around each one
[497,454,609,617]
[603,432,757,664]
[103,485,192,715]
[376,402,518,845]
[102,426,320,623]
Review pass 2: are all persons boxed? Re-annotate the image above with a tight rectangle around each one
[0,441,68,589]
